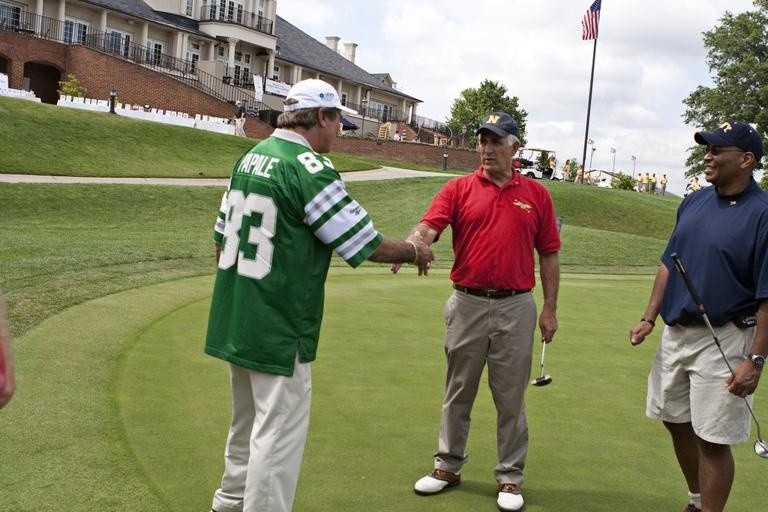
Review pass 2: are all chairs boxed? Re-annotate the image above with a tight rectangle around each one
[377,121,391,139]
[0,13,51,42]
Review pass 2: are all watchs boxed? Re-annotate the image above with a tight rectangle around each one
[746,352,765,368]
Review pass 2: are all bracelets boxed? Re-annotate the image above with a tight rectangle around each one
[406,240,419,266]
[638,318,655,327]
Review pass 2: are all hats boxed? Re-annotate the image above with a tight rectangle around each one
[281,77,359,117]
[235,100,242,105]
[473,111,521,142]
[693,118,763,160]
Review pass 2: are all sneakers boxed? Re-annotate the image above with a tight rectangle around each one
[494,482,527,511]
[682,503,702,512]
[412,464,462,496]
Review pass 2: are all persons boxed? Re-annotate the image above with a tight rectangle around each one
[627,118,767,511]
[200,77,435,511]
[388,112,561,511]
[632,170,668,195]
[233,101,248,137]
[393,131,401,141]
[574,165,584,184]
[549,156,556,180]
[683,177,702,198]
[562,159,570,181]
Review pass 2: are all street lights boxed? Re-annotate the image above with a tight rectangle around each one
[631,154,637,179]
[461,124,466,148]
[361,96,368,135]
[610,147,617,174]
[588,137,597,179]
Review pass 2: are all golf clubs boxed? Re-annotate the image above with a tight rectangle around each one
[671,252,768,458]
[531,215,563,386]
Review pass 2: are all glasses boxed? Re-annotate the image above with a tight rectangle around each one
[704,145,748,156]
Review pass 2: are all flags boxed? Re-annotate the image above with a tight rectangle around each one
[580,1,602,41]
